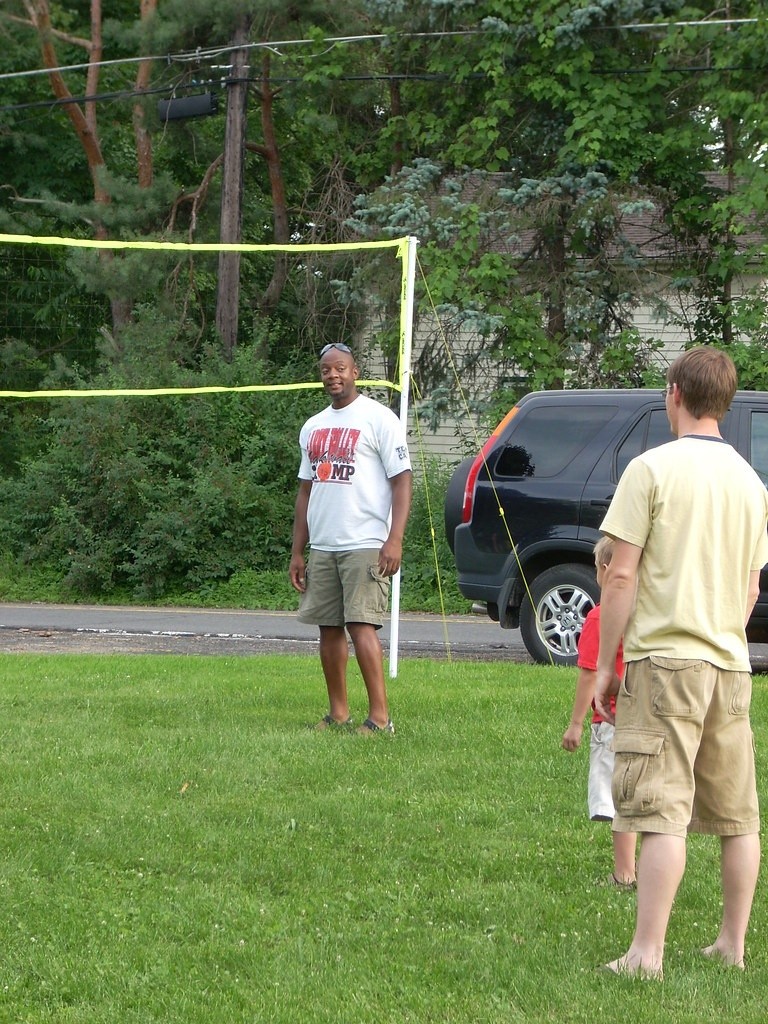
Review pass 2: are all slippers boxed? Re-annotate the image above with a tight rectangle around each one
[585,964,618,977]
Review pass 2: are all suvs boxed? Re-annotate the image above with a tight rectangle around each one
[443,389,768,667]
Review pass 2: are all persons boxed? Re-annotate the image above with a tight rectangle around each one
[594,346,768,983]
[562,537,636,890]
[288,341,415,737]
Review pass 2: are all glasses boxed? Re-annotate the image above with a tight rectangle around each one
[660,384,680,399]
[318,342,356,360]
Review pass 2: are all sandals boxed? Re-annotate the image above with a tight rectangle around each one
[310,714,353,733]
[349,719,395,737]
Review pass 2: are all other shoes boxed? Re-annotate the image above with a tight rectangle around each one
[606,869,639,894]
